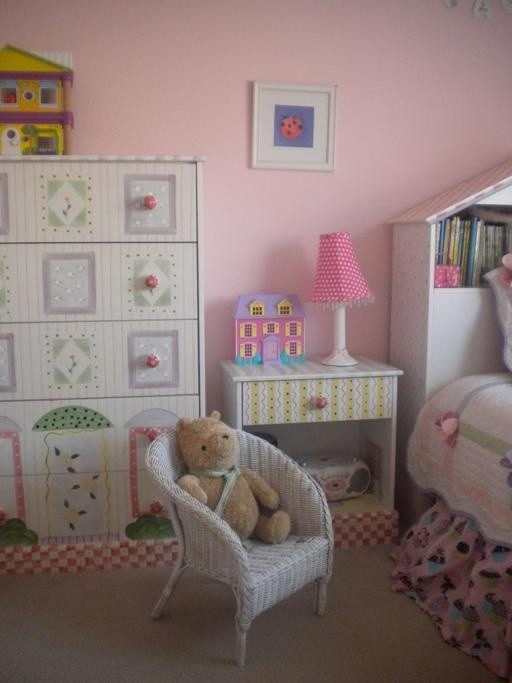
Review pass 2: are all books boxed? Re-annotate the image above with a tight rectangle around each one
[435,215,512,285]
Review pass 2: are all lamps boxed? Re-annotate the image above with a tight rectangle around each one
[306,231,376,367]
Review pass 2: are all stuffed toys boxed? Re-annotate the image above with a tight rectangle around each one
[174,408,293,545]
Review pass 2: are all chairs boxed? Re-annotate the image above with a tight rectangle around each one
[143,427,333,668]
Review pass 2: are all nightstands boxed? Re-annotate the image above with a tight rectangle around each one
[219,356,405,552]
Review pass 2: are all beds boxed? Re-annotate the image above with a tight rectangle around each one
[389,371,512,630]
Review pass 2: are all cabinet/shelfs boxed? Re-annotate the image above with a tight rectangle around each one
[0,154,207,577]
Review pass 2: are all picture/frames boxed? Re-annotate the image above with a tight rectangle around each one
[250,80,337,173]
